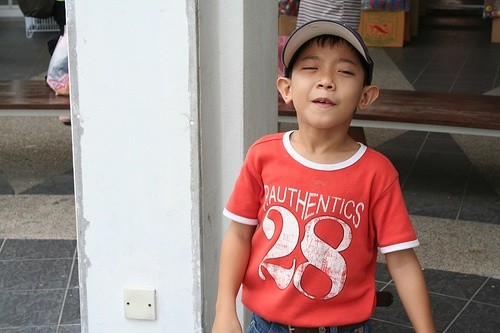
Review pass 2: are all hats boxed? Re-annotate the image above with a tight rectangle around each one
[281,19,374,87]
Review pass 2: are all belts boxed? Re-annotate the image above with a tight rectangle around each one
[262,291,394,333]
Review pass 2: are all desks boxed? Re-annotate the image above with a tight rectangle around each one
[359,10,410,47]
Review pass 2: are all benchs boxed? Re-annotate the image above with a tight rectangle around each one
[422,3,486,27]
[0,80,500,137]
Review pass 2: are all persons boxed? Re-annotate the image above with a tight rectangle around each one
[296,0,361,34]
[210,19,438,333]
